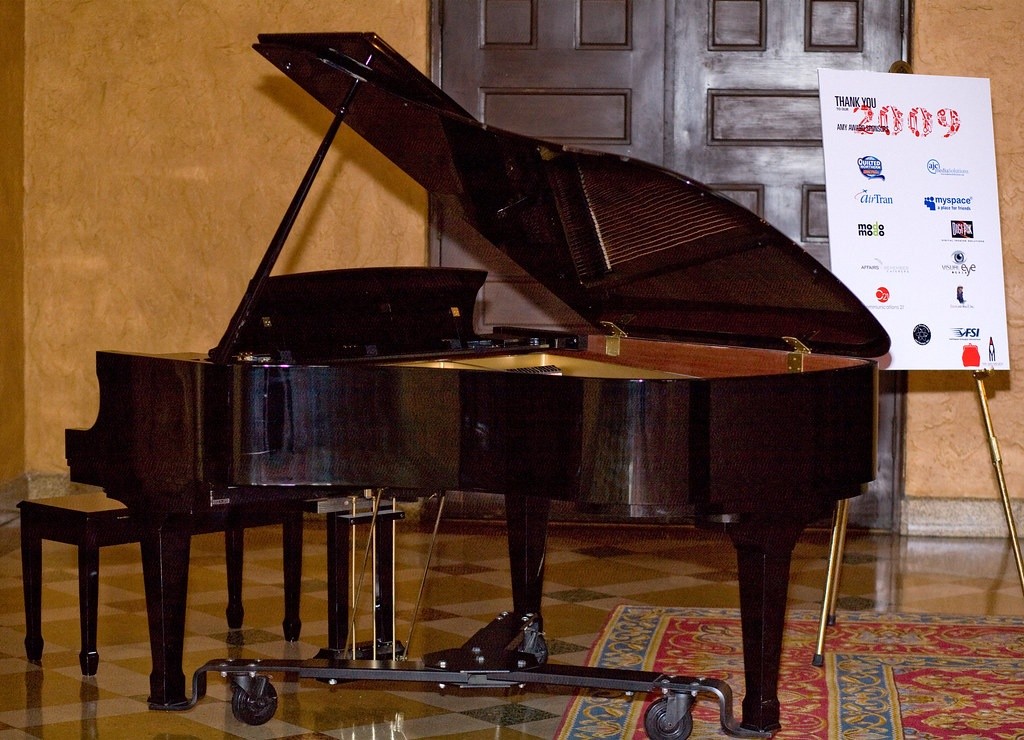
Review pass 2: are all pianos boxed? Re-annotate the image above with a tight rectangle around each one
[65,32,891,732]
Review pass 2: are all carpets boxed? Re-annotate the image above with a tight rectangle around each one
[554,603,1024,740]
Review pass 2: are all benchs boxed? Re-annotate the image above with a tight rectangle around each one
[15,492,305,676]
